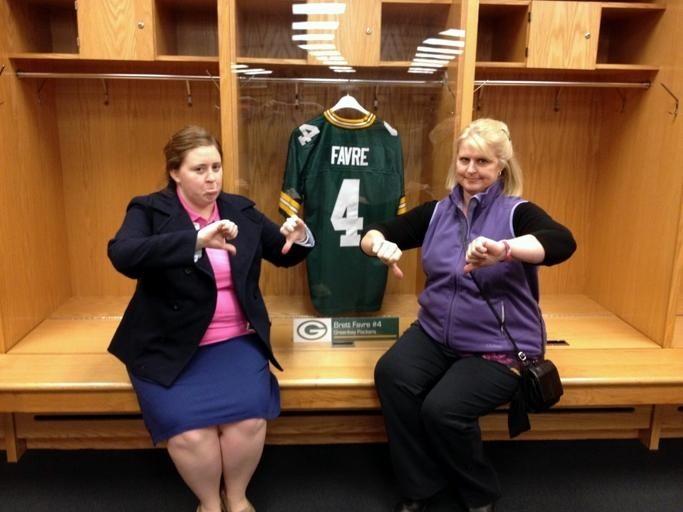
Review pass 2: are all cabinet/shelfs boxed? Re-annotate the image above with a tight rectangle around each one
[0,1,682,461]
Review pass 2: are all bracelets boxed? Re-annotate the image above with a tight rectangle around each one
[499,239,513,262]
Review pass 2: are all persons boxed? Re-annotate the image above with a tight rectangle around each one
[359,118,576,512]
[107,126,315,512]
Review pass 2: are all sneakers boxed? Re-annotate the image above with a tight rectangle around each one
[195,488,256,512]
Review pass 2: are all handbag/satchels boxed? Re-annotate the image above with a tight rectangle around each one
[514,354,565,415]
